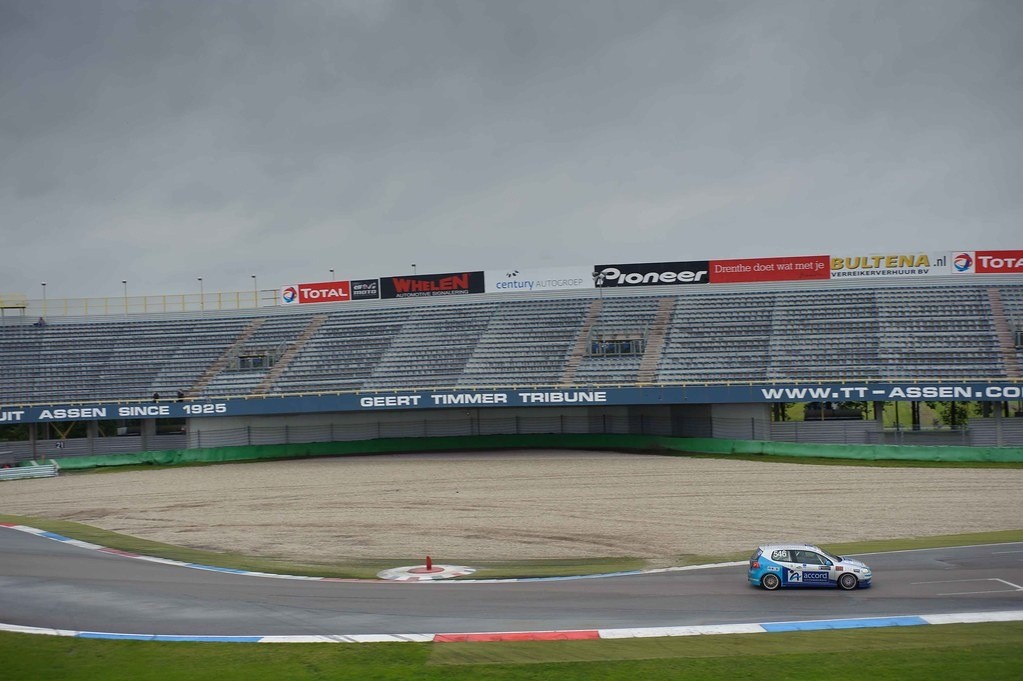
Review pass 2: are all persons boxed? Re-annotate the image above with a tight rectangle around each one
[153,392,160,403]
[178,389,184,402]
[39,317,44,324]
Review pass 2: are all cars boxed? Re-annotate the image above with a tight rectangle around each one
[748,544,873,591]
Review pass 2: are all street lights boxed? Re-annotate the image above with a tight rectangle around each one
[41,281,47,318]
[412,263,418,275]
[251,274,257,307]
[198,276,205,312]
[122,279,129,315]
[329,268,335,282]
